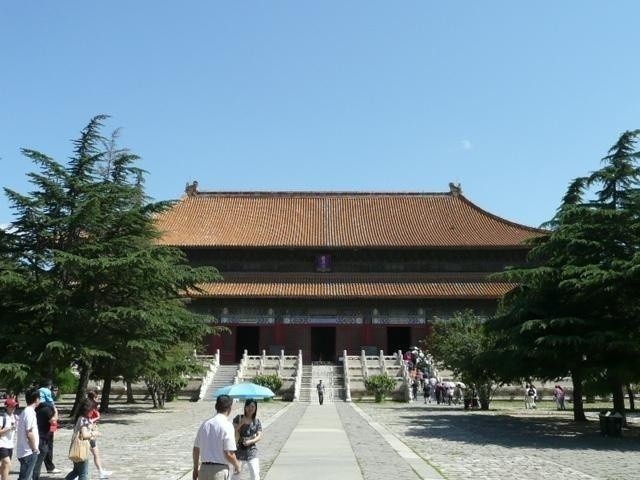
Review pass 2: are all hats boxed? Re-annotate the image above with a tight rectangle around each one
[5,398,18,408]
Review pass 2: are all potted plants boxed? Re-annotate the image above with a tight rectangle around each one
[364,371,398,403]
[254,374,284,402]
[161,376,188,402]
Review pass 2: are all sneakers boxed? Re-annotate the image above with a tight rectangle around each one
[100,471,114,478]
[47,469,62,473]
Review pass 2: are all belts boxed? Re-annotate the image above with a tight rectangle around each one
[202,462,223,466]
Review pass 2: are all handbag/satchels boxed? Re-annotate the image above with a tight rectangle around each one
[69,438,90,463]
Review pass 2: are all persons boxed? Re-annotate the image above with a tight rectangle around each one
[234,399,262,480]
[0,380,112,480]
[405,345,479,410]
[193,395,241,480]
[317,380,326,405]
[553,386,566,410]
[525,384,537,409]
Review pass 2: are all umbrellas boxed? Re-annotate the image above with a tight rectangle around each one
[213,379,277,430]
